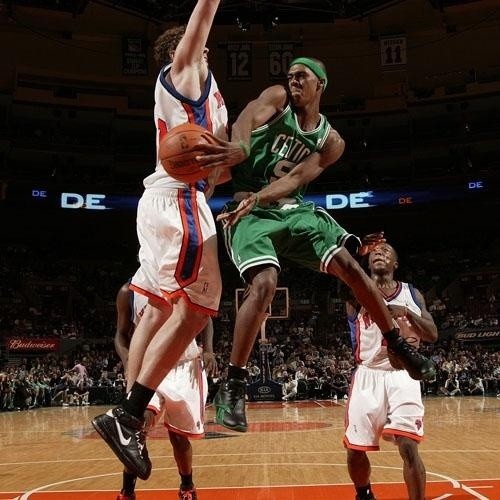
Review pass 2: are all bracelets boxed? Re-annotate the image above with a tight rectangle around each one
[235,137,253,159]
[254,189,262,209]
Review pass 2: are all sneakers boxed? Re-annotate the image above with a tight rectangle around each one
[116,489,136,500]
[178,482,198,500]
[386,336,436,380]
[91,404,153,480]
[213,374,249,432]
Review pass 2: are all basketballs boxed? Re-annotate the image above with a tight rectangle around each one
[159,123,221,183]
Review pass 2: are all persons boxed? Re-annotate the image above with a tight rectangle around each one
[1,241,500,416]
[191,52,443,431]
[112,258,217,500]
[334,227,440,499]
[90,0,232,485]
